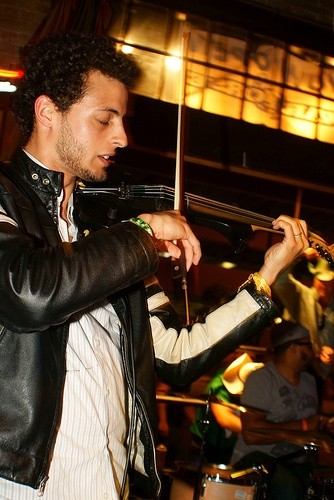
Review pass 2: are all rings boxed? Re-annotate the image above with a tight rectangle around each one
[294,233,301,236]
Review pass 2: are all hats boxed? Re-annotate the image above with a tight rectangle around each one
[271,321,308,349]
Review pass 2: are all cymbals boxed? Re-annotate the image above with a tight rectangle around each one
[247,427,334,454]
[155,394,273,414]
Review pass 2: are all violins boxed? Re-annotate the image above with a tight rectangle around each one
[73,184,334,273]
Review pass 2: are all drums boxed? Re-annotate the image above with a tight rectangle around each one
[168,469,201,500]
[308,466,334,495]
[193,463,259,500]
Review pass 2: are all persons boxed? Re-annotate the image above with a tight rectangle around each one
[156,247,334,474]
[0,23,310,500]
[229,320,334,500]
